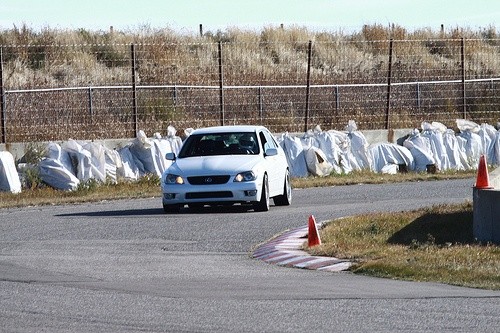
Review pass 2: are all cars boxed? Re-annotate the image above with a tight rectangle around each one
[160,124,292,213]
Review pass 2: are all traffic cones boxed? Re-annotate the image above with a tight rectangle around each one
[471,155,496,189]
[308,214,321,247]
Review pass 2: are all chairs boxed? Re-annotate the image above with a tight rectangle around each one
[199,140,224,154]
[229,144,259,155]
